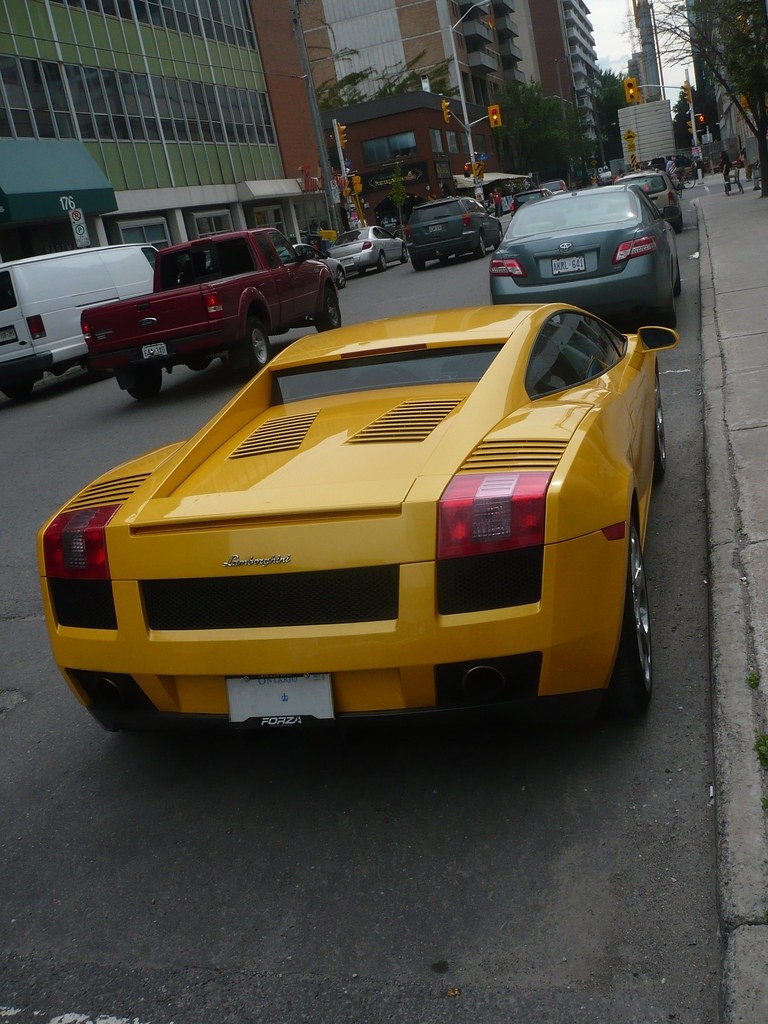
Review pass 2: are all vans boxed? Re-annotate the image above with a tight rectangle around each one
[0,243,160,400]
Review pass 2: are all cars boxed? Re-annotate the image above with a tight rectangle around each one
[325,226,408,276]
[537,179,569,195]
[405,196,503,270]
[292,243,347,289]
[489,184,680,335]
[510,189,554,216]
[613,169,684,235]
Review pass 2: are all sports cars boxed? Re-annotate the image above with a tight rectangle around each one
[36,302,682,737]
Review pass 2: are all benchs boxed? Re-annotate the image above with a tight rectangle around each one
[723,168,743,195]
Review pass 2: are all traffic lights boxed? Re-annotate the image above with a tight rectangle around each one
[342,181,352,198]
[624,78,638,103]
[686,121,693,134]
[462,165,470,178]
[487,105,502,128]
[441,99,451,123]
[352,175,363,193]
[699,116,705,122]
[338,123,347,149]
[680,83,691,103]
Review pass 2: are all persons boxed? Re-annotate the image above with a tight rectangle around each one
[719,150,732,192]
[665,155,684,189]
[740,147,753,181]
[488,188,503,218]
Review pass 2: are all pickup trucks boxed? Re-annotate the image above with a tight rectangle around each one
[79,228,342,400]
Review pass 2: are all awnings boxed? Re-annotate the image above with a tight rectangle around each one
[451,171,530,188]
